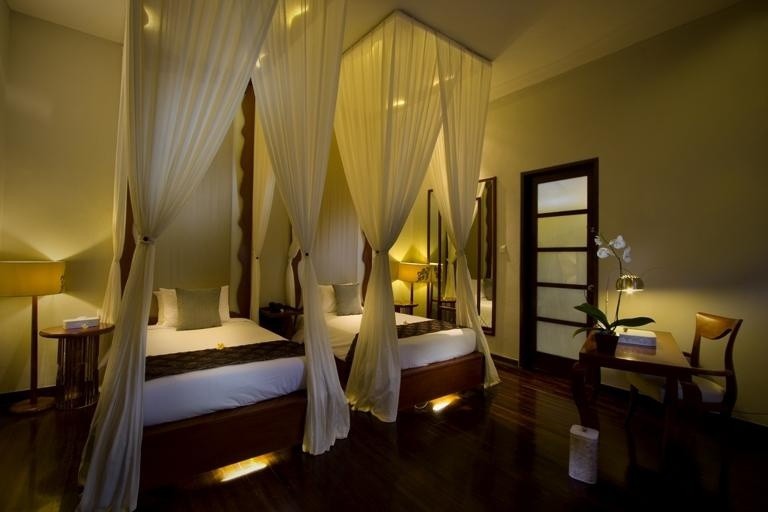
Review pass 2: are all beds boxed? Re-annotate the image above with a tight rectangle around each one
[292,312,490,422]
[138,317,308,502]
[473,297,493,328]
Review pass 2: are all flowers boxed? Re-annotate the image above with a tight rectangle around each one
[574,231,655,339]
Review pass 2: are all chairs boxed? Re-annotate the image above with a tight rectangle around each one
[625,312,743,487]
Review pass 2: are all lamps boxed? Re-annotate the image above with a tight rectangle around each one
[0,261,66,415]
[605,267,644,319]
[430,262,443,282]
[399,263,430,305]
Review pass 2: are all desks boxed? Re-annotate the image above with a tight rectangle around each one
[395,304,418,315]
[433,297,456,319]
[260,306,302,337]
[570,329,701,428]
[39,322,115,409]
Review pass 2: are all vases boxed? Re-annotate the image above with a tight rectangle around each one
[593,331,618,354]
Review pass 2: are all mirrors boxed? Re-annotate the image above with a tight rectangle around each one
[427,177,497,335]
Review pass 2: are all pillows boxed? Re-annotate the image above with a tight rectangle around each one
[482,278,492,300]
[332,283,363,316]
[152,285,230,330]
[318,283,361,313]
[472,279,486,297]
